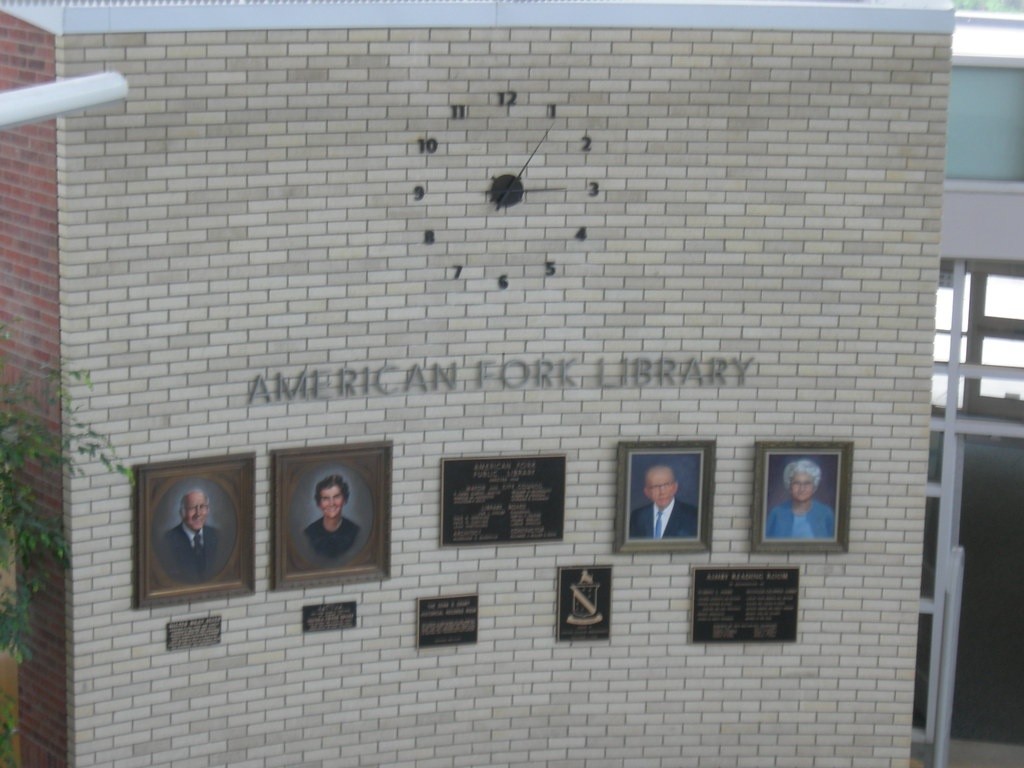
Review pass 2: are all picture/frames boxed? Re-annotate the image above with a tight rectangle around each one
[752,441,855,555]
[271,439,392,592]
[131,451,256,611]
[613,438,717,555]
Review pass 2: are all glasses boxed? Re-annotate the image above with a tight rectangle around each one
[648,482,675,490]
[790,480,813,487]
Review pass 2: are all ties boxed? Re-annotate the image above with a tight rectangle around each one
[655,511,662,540]
[193,529,201,550]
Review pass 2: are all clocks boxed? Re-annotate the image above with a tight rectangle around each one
[412,89,601,289]
